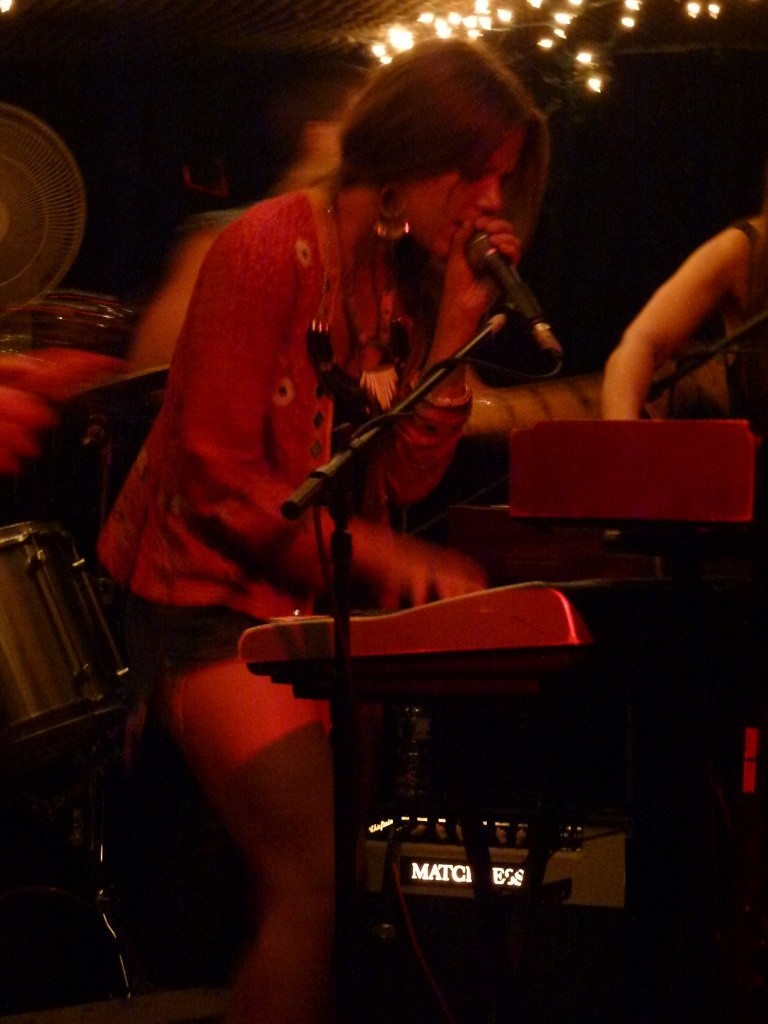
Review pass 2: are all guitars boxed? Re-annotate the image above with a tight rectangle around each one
[452,348,725,439]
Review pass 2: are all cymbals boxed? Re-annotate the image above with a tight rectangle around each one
[50,362,172,419]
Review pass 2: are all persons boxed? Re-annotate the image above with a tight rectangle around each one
[94,38,554,1023]
[601,214,768,422]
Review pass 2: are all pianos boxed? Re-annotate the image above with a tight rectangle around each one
[236,574,768,708]
[505,418,767,525]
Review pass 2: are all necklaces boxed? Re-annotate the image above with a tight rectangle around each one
[328,183,382,350]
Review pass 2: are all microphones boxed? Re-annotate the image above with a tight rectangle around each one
[463,229,564,362]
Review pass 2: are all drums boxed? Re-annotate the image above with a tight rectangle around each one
[0,288,138,362]
[1,516,135,754]
